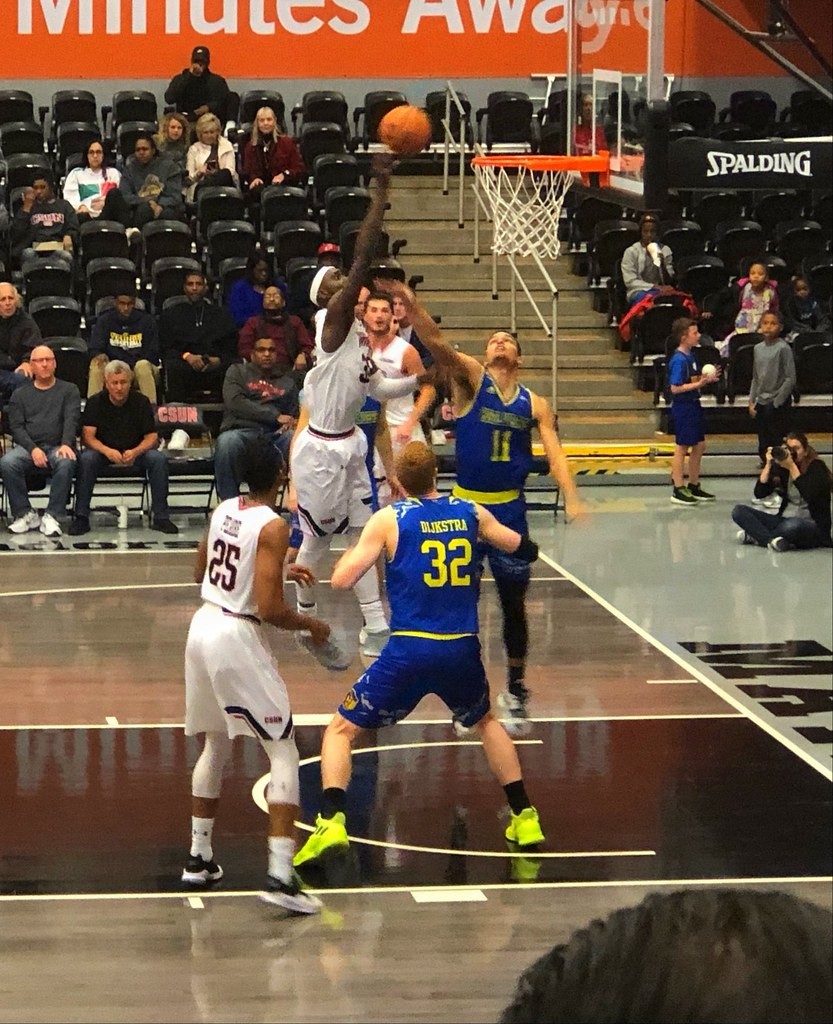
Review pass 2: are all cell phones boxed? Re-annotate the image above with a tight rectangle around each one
[200,355,211,365]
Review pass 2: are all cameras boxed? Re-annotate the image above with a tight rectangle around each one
[770,443,798,461]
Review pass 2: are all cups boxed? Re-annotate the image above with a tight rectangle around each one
[647,241,664,261]
[116,505,129,528]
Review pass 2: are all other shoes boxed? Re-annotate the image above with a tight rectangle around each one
[770,536,795,551]
[751,492,783,508]
[152,518,179,533]
[453,720,478,736]
[68,514,91,535]
[497,686,532,711]
[736,530,758,545]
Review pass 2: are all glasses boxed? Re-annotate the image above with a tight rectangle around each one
[31,357,55,363]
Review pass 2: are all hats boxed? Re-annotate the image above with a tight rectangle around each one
[640,212,660,224]
[192,46,210,61]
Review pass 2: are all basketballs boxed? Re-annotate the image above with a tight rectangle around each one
[378,105,433,156]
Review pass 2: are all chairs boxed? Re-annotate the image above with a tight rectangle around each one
[1,89,833,531]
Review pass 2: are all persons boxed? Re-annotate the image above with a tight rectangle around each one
[291,441,545,869]
[732,431,833,551]
[618,211,833,358]
[10,47,311,267]
[669,318,721,506]
[289,157,578,737]
[749,309,797,469]
[181,436,329,916]
[574,96,607,154]
[0,242,341,533]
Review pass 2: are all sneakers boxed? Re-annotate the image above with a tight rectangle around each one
[687,482,716,502]
[181,850,224,883]
[359,626,390,658]
[39,513,63,537]
[506,840,541,880]
[670,486,699,505]
[292,812,350,869]
[505,805,546,846]
[257,868,323,914]
[294,627,351,670]
[7,508,41,534]
[259,913,320,956]
